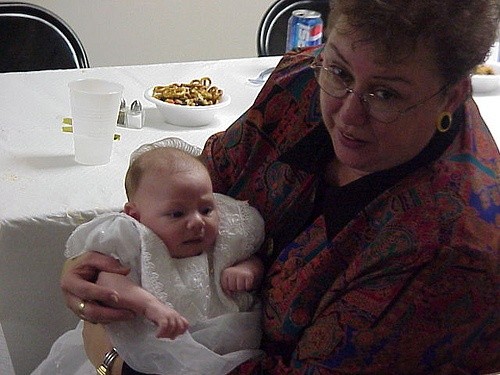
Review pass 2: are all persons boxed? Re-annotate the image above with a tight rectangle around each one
[60,0,500,375]
[60,137,272,375]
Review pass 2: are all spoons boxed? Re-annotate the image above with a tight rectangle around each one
[248,67,275,84]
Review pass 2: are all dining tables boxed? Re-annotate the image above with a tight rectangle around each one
[0,43,500,375]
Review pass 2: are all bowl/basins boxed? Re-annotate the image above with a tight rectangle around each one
[469,61,500,93]
[144,83,231,126]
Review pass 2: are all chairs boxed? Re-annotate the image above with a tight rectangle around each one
[0,0,90,74]
[257,0,331,57]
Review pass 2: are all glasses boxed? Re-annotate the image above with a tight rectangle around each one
[307,42,458,124]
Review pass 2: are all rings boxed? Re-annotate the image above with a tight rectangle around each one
[79,300,87,315]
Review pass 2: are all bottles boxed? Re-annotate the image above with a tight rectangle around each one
[117,99,144,129]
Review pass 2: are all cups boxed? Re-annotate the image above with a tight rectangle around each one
[69,78,124,165]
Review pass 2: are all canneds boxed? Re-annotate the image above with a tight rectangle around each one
[285,9,323,53]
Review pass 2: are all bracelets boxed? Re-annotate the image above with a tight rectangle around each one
[95,347,121,375]
